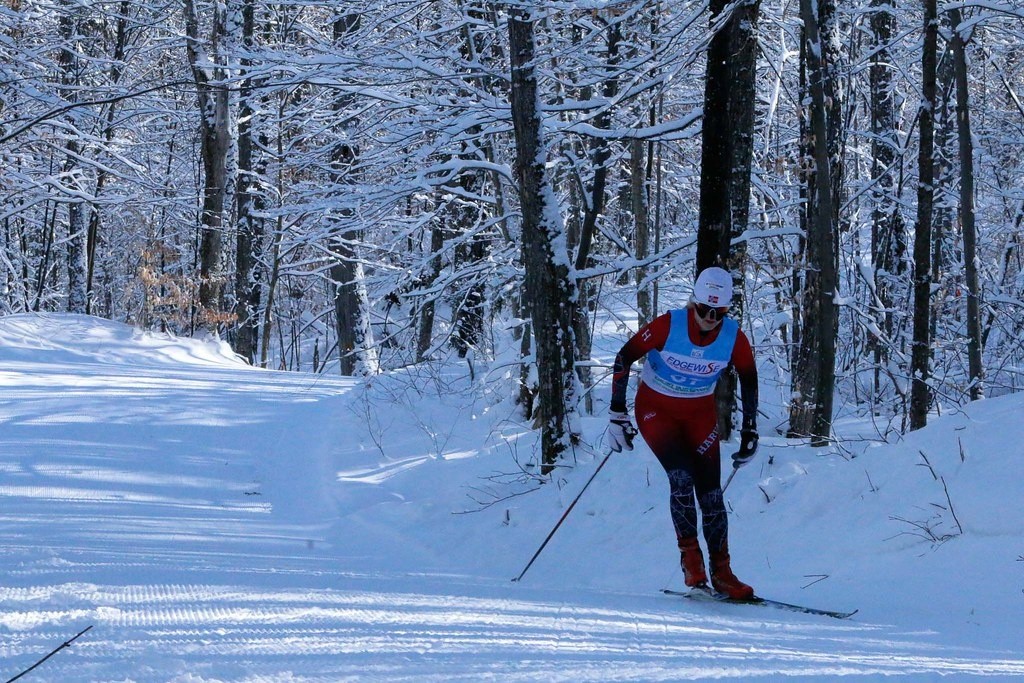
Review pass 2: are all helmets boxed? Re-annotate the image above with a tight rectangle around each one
[693,267,732,308]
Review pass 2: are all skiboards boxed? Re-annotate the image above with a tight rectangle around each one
[658,586,861,621]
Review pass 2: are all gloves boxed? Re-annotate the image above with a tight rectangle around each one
[731,419,759,469]
[608,406,638,453]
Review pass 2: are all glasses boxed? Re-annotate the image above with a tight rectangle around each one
[694,303,727,322]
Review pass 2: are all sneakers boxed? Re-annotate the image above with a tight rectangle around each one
[709,555,754,599]
[679,542,707,586]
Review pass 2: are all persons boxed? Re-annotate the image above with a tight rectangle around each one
[609,268,760,602]
